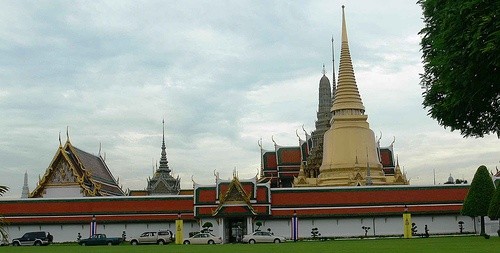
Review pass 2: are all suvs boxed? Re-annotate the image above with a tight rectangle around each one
[129,229,175,245]
[12,231,53,246]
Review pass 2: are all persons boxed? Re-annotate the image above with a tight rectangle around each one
[425,224,430,238]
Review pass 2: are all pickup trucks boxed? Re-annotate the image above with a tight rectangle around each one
[79,234,123,246]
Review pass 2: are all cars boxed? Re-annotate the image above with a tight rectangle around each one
[243,231,286,244]
[183,233,222,245]
